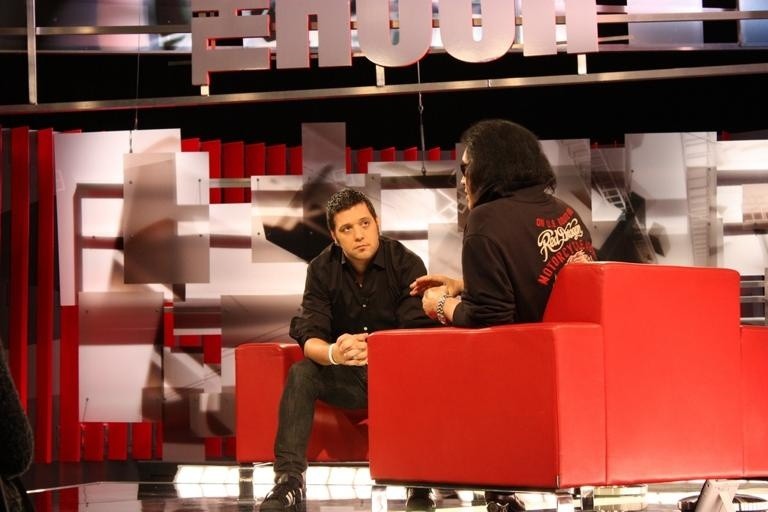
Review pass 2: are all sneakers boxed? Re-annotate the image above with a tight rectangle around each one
[405,485,435,511]
[484,491,525,512]
[260,472,306,512]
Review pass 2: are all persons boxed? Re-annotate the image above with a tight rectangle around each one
[260,189,436,512]
[410,119,598,328]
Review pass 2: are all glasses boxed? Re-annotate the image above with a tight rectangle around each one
[460,164,469,176]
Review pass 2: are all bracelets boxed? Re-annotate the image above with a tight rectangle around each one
[329,343,338,365]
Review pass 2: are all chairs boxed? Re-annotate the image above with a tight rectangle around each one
[235,341,369,483]
[368,261,768,512]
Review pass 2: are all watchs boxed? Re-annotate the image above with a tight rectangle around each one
[437,294,448,325]
